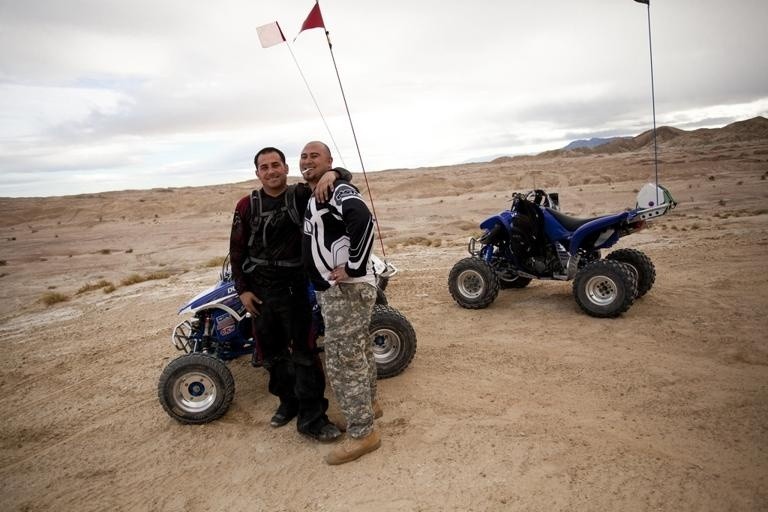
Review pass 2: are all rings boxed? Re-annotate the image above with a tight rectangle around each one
[334,273,336,277]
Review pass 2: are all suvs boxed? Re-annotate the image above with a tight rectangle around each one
[158,247,418,426]
[447,187,678,316]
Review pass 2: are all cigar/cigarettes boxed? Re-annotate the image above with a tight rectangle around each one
[301,168,311,175]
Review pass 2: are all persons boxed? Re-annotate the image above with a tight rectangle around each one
[230,146,354,442]
[299,141,385,465]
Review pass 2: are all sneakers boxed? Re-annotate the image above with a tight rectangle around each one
[319,423,342,441]
[328,432,381,465]
[270,414,288,427]
[326,401,383,430]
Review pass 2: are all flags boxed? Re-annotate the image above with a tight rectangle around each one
[256,21,287,50]
